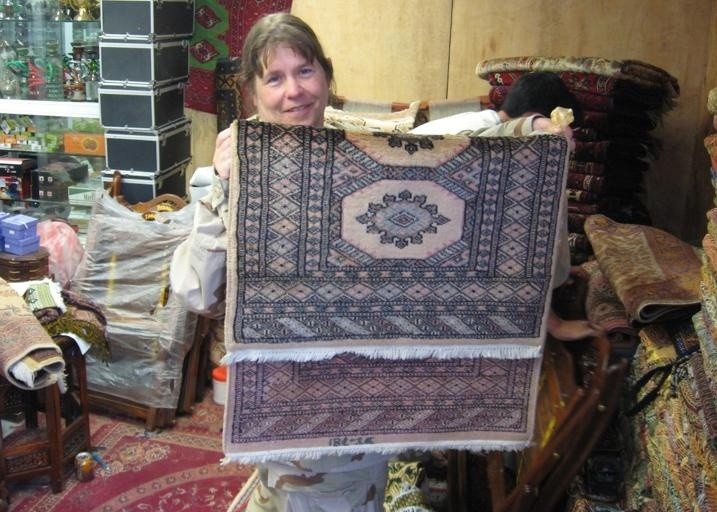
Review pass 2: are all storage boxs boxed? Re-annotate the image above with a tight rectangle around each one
[99,87,187,131]
[98,168,188,212]
[99,37,189,89]
[105,118,193,179]
[99,0,198,41]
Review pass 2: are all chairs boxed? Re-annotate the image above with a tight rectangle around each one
[464,330,630,512]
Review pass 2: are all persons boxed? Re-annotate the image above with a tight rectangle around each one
[169,10,572,511]
[546,297,606,342]
[407,69,568,134]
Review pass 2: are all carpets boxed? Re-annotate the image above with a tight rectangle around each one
[4,389,264,512]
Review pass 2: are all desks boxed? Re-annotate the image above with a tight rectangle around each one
[1,275,115,505]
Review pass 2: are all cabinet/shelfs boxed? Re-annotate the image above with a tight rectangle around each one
[1,0,105,287]
